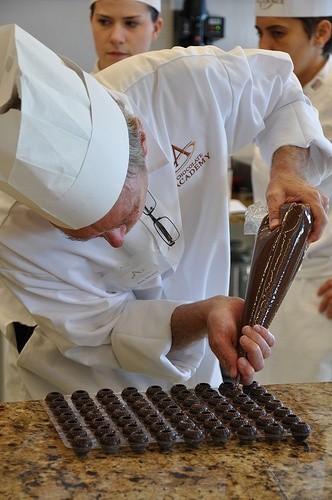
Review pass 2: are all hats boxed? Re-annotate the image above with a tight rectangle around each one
[1,23,130,230]
[89,0,161,14]
[255,0,332,17]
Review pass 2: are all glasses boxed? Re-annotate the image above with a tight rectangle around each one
[143,190,180,246]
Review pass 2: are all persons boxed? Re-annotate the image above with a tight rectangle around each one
[89,0,164,73]
[0,24,332,402]
[249,0,331,383]
